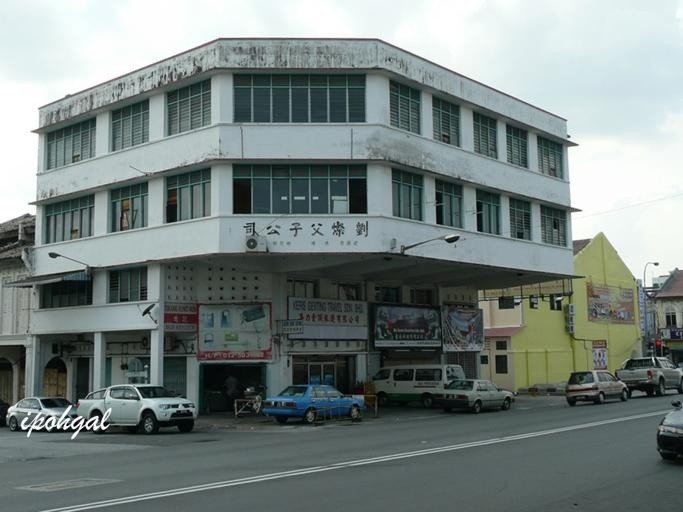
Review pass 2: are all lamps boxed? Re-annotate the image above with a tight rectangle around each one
[48,252,90,276]
[401,234,461,254]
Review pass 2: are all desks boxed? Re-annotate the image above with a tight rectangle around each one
[340,395,378,417]
[234,399,273,421]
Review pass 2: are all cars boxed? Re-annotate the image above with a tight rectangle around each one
[431,377,515,414]
[6,397,79,431]
[0,400,10,427]
[263,384,366,424]
[565,370,630,407]
[310,373,332,386]
[657,399,683,464]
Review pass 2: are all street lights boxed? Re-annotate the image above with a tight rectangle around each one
[643,261,659,354]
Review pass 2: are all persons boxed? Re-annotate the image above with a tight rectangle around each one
[223,372,241,410]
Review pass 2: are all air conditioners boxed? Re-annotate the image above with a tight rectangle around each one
[565,303,575,335]
[246,235,267,252]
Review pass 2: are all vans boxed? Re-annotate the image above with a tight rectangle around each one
[373,364,467,406]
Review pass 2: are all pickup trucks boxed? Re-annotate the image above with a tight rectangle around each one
[614,357,683,397]
[76,383,197,434]
